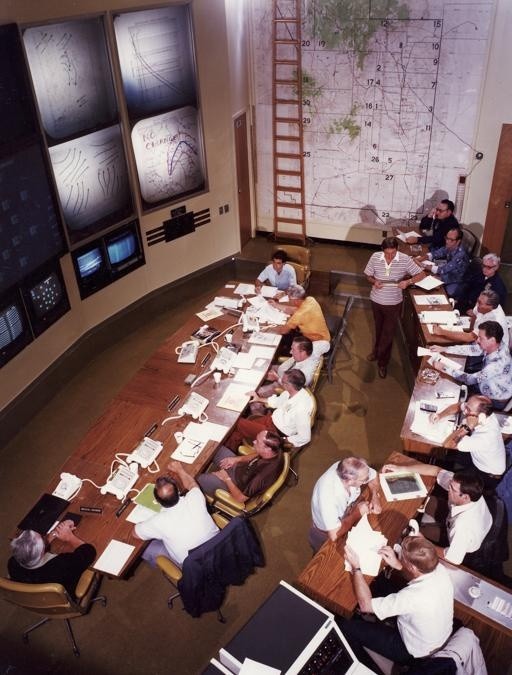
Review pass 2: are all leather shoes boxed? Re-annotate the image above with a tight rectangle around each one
[367,352,377,362]
[377,365,387,378]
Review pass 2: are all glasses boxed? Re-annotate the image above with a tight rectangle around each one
[482,263,497,270]
[436,208,450,213]
[445,236,460,242]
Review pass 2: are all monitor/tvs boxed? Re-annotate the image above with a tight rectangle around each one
[104,220,144,278]
[0,288,33,369]
[71,240,108,297]
[22,260,71,338]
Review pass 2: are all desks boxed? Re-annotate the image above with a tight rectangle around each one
[291,450,436,621]
[15,294,303,577]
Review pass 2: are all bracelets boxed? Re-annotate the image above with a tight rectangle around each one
[409,279,413,285]
[224,476,232,483]
[352,568,361,573]
[460,424,472,437]
[283,436,286,443]
[51,530,57,537]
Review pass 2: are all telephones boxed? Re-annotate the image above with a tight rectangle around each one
[393,544,402,561]
[460,385,468,402]
[462,413,487,426]
[181,340,200,347]
[408,519,419,537]
[241,325,260,333]
[454,309,460,318]
[60,471,81,485]
[432,213,435,220]
[449,298,455,308]
[178,406,199,419]
[101,484,124,500]
[125,454,148,469]
[238,297,247,308]
[210,363,230,374]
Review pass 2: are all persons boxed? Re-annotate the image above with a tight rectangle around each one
[255,250,296,300]
[382,464,493,566]
[432,290,509,366]
[429,321,510,412]
[195,430,285,504]
[429,395,506,491]
[413,227,469,298]
[406,200,460,253]
[363,238,426,378]
[7,520,97,605]
[308,457,382,556]
[131,461,221,572]
[345,537,454,663]
[455,253,507,318]
[223,369,314,455]
[259,284,331,356]
[250,336,320,416]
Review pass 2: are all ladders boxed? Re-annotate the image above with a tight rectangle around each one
[270,0,307,245]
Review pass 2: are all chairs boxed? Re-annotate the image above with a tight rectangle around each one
[0,567,107,658]
[204,446,290,517]
[156,512,251,619]
[239,296,356,482]
[270,244,312,295]
[390,224,512,675]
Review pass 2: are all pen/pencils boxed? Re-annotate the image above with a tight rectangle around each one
[193,442,201,449]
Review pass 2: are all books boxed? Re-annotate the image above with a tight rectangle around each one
[190,323,220,345]
